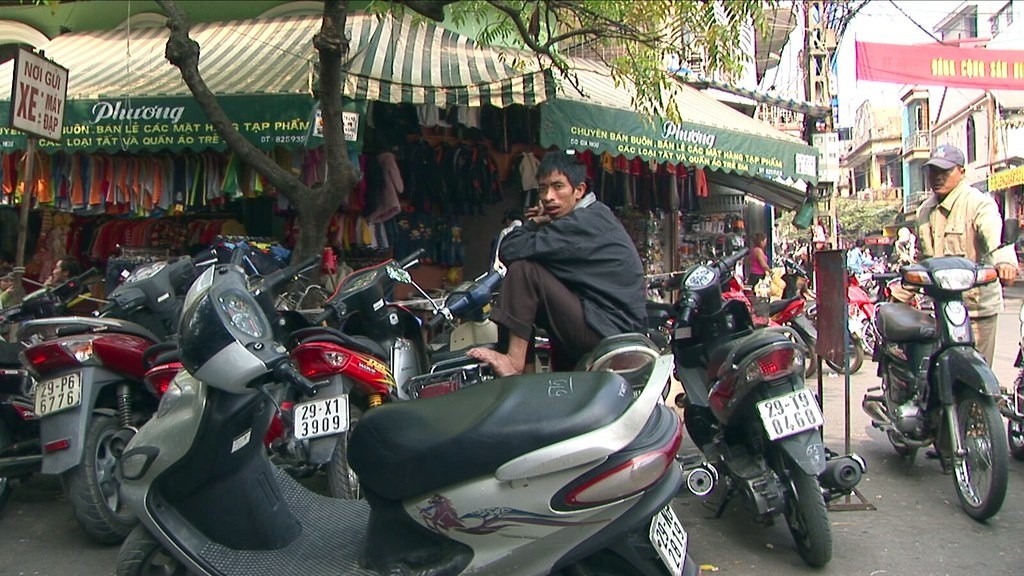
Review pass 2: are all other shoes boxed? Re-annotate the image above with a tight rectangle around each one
[926,447,951,459]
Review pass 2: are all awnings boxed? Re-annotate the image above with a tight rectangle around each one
[0,12,556,155]
[540,53,832,213]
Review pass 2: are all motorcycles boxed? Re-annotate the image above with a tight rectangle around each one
[861,218,1020,521]
[667,244,869,571]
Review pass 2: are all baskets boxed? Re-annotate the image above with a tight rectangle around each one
[725,296,770,327]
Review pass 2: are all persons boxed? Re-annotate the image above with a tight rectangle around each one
[0,255,98,315]
[747,233,772,295]
[463,151,649,379]
[915,146,1020,459]
[793,227,917,287]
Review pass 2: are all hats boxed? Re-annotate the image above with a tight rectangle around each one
[502,208,522,223]
[921,146,964,170]
[898,227,910,243]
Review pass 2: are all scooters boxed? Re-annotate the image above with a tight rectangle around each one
[113,249,705,576]
[998,328,1023,461]
[0,239,935,548]
